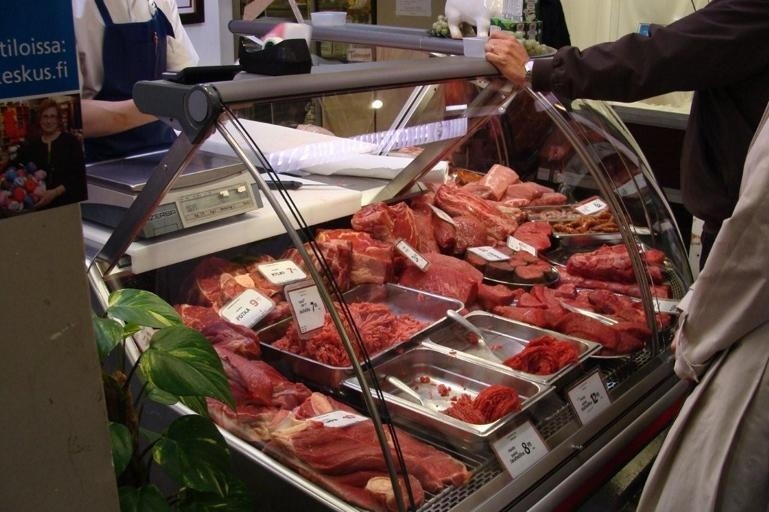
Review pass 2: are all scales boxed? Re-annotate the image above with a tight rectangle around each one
[81,149,264,239]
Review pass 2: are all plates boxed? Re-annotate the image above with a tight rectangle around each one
[483,263,560,287]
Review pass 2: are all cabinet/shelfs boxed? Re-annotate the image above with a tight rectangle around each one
[83,16,696,510]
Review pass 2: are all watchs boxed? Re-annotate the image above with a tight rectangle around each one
[524,59,535,81]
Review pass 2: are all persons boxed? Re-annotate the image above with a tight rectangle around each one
[9,98,88,211]
[625,100,768,512]
[71,0,230,167]
[482,1,769,270]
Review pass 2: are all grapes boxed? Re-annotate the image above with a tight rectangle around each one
[511,30,547,56]
[431,14,449,37]
